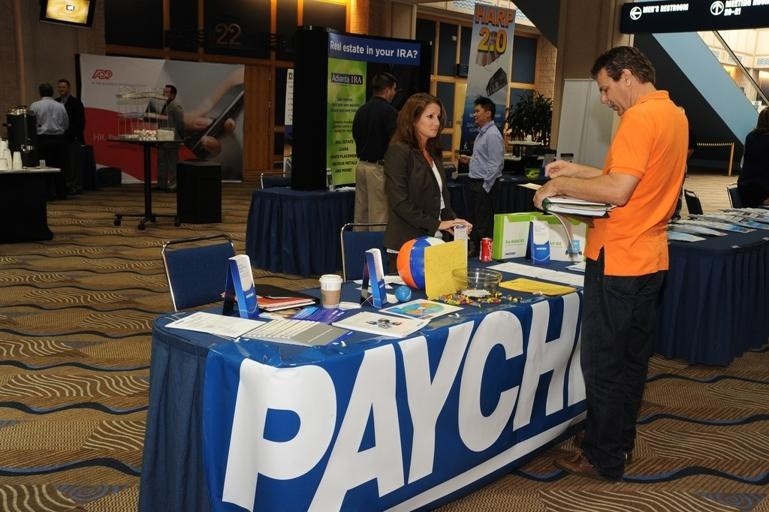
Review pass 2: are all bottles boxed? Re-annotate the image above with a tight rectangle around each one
[13,152,22,170]
[40,160,45,169]
[527,136,533,143]
[539,150,574,182]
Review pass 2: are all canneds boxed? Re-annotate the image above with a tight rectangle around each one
[480,237,494,263]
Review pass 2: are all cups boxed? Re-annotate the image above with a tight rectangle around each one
[319,274,343,309]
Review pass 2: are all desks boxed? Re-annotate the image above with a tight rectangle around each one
[106,136,185,229]
[136,258,591,512]
[0,165,61,245]
[246,184,467,276]
[456,174,551,234]
[657,222,769,366]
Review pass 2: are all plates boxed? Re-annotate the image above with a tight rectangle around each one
[122,130,175,141]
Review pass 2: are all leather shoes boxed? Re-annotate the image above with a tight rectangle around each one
[552,431,632,484]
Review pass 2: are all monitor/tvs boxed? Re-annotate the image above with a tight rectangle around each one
[38,0,97,32]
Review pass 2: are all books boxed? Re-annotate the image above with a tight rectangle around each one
[541,194,612,218]
[220,286,313,308]
[266,300,316,313]
[330,311,431,339]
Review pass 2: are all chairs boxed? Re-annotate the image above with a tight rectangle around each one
[341,222,390,283]
[161,234,236,312]
[260,172,289,189]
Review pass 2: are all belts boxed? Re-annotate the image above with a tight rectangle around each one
[359,156,385,166]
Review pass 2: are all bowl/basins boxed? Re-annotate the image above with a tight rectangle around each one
[452,269,502,298]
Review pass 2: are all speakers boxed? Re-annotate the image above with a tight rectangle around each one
[177,160,223,222]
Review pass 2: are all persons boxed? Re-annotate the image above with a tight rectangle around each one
[532,47,691,477]
[155,83,187,191]
[737,104,768,206]
[349,72,400,233]
[51,79,88,196]
[382,90,472,275]
[670,130,698,222]
[455,96,506,258]
[182,70,246,158]
[29,84,70,200]
